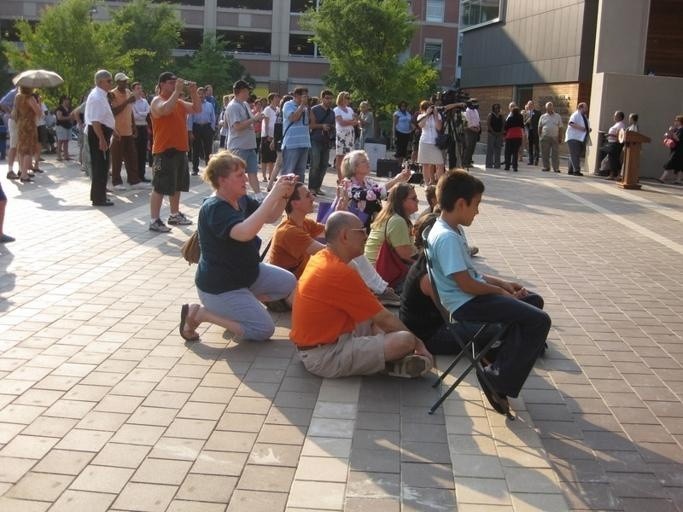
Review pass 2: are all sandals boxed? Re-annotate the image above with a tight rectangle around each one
[179,304,200,340]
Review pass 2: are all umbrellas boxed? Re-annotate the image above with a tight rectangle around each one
[12,69,64,88]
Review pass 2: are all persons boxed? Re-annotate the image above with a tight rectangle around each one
[0,86,23,179]
[486,103,505,169]
[426,168,552,415]
[36,95,58,152]
[503,107,524,172]
[186,87,216,176]
[179,91,185,101]
[84,70,115,206]
[656,115,683,184]
[74,101,83,165]
[262,182,401,311]
[281,88,312,184]
[538,102,563,173]
[393,101,412,161]
[188,146,192,162]
[399,213,548,355]
[333,91,360,185]
[313,97,320,106]
[250,100,262,169]
[267,96,294,193]
[219,97,230,148]
[334,150,411,236]
[15,85,42,183]
[417,100,444,191]
[146,85,160,167]
[564,102,592,176]
[261,93,281,182]
[31,93,50,161]
[179,152,299,342]
[409,100,428,170]
[357,101,375,149]
[131,82,152,183]
[306,96,313,112]
[260,98,268,112]
[604,111,626,180]
[462,98,481,168]
[289,211,434,378]
[205,85,218,155]
[55,95,74,161]
[109,73,146,189]
[149,72,202,232]
[501,102,516,165]
[617,113,640,182]
[308,90,336,197]
[414,185,478,256]
[518,105,529,161]
[523,100,542,166]
[363,182,420,296]
[141,91,146,99]
[247,94,257,114]
[0,181,16,242]
[225,96,235,151]
[444,113,458,173]
[227,81,261,194]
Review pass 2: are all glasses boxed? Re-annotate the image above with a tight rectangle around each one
[345,97,349,99]
[351,227,367,234]
[408,195,417,201]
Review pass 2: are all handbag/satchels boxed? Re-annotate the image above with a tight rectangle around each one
[435,131,450,150]
[664,138,675,147]
[181,231,201,264]
[258,238,304,312]
[376,214,408,283]
[278,121,294,151]
[314,198,369,245]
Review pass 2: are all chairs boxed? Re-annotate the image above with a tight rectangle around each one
[421,223,518,423]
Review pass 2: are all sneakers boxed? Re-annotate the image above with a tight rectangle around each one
[132,182,146,190]
[168,214,191,225]
[93,200,113,206]
[317,191,325,195]
[0,235,15,243]
[573,171,583,176]
[381,355,431,378]
[568,171,573,175]
[309,188,316,195]
[542,168,548,171]
[470,247,478,255]
[381,288,401,307]
[7,171,19,179]
[477,357,498,375]
[555,169,560,173]
[113,184,127,191]
[149,219,171,233]
[477,369,509,414]
[605,176,613,180]
[20,177,35,182]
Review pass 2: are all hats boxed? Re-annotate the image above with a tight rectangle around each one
[233,80,253,90]
[114,73,130,82]
[158,72,176,87]
